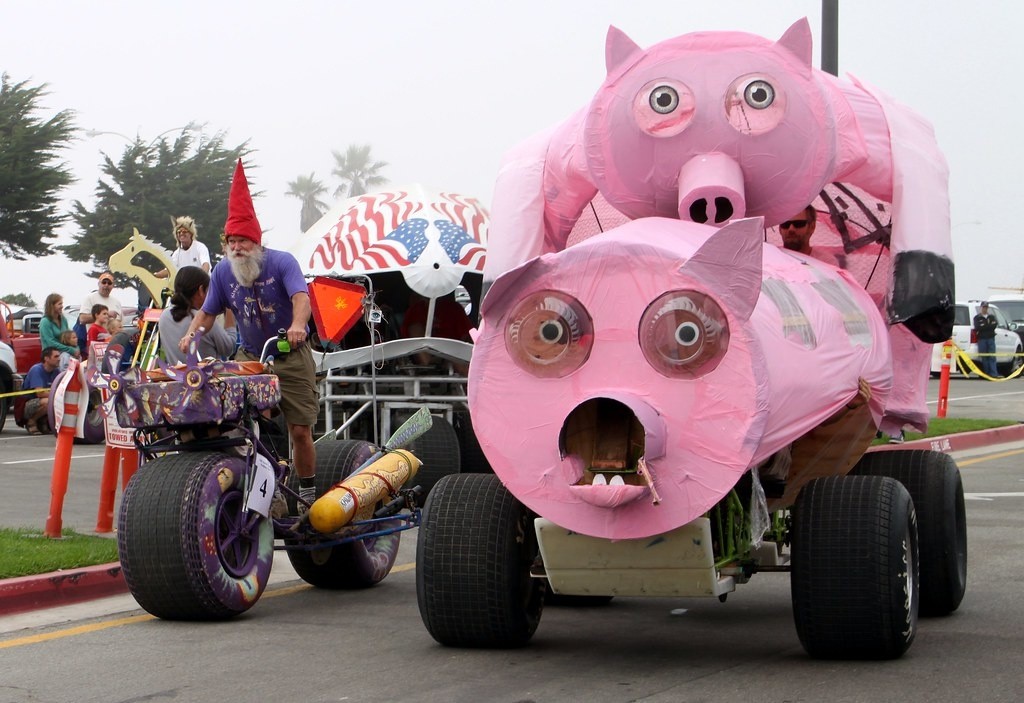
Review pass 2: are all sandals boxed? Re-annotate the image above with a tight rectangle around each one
[25,419,42,435]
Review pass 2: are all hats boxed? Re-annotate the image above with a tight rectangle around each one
[170,215,198,247]
[224,158,262,246]
[99,273,114,285]
[980,301,989,308]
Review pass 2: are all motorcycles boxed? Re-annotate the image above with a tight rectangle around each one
[118,329,423,622]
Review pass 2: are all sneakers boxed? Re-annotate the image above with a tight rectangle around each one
[888,430,905,443]
[297,485,315,516]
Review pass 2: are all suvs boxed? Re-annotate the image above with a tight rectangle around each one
[931,300,1023,378]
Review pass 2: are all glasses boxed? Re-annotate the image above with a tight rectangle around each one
[177,230,189,234]
[101,281,112,285]
[779,219,811,230]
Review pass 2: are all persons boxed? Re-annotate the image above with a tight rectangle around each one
[0,214,239,437]
[974,301,1005,380]
[178,157,319,523]
